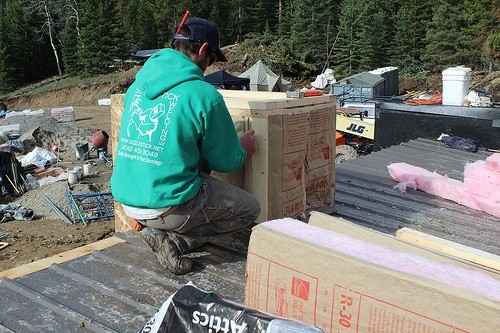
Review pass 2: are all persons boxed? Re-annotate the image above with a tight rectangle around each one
[109,17,261,276]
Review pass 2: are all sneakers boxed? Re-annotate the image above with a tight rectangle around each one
[139,226,191,275]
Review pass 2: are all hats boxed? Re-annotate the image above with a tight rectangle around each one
[173,18,228,62]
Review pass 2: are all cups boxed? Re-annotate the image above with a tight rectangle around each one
[74,167,82,178]
[68,172,77,184]
[83,164,91,175]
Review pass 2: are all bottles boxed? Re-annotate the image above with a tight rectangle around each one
[90,131,109,148]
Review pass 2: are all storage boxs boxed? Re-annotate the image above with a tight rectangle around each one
[441,65,473,106]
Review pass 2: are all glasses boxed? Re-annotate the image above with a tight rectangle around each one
[198,42,215,66]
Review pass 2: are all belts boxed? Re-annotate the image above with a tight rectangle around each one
[160,206,179,217]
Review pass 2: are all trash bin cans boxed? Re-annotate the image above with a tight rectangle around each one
[442,65,472,106]
